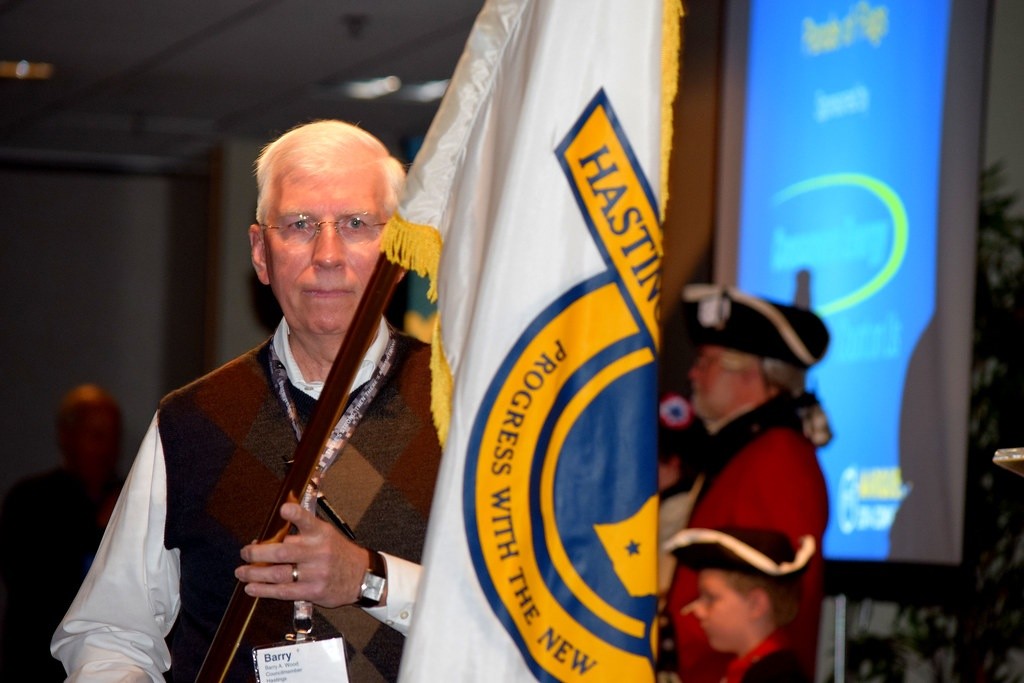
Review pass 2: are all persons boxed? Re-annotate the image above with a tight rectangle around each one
[675,277,835,681]
[1,383,137,681]
[40,115,448,683]
[666,525,819,682]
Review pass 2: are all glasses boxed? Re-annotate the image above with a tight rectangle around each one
[258,210,387,244]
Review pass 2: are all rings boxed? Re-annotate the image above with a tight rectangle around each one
[291,564,300,584]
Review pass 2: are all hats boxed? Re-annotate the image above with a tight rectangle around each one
[680,282,832,367]
[666,519,816,586]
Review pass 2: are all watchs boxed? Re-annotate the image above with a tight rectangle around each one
[353,547,387,610]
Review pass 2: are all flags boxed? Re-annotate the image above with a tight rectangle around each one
[386,3,686,683]
